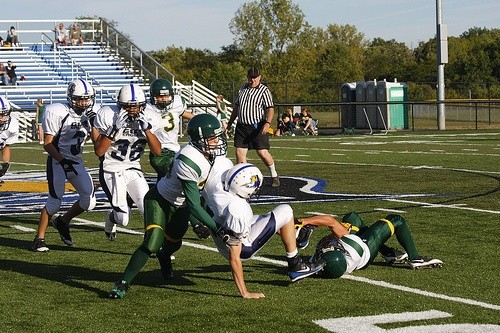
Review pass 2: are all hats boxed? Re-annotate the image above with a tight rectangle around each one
[248,67,260,79]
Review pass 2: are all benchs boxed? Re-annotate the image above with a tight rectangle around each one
[0,41,150,109]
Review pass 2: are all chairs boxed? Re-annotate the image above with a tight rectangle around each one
[307,119,319,135]
[280,121,291,135]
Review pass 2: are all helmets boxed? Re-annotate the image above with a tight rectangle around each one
[66,79,174,122]
[0,96,11,130]
[224,162,264,199]
[186,113,228,157]
[317,251,347,279]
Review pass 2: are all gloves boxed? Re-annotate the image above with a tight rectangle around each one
[293,218,305,229]
[0,163,10,178]
[59,157,80,179]
[135,109,152,132]
[211,221,240,251]
[85,111,97,128]
[105,106,129,141]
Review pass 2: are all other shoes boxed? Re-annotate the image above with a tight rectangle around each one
[291,133,296,137]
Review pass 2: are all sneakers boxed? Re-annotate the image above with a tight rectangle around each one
[271,175,281,188]
[104,211,117,241]
[295,254,314,262]
[155,269,175,286]
[410,254,444,270]
[297,227,314,250]
[50,214,73,245]
[32,239,50,252]
[155,247,176,261]
[384,247,409,265]
[287,263,326,282]
[193,223,211,239]
[109,280,130,299]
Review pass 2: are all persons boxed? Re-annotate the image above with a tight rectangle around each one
[277,108,297,137]
[35,98,46,146]
[30,79,444,301]
[69,23,85,46]
[7,25,19,47]
[0,61,20,85]
[215,94,229,140]
[51,23,68,46]
[0,96,21,178]
[226,67,281,188]
[297,110,318,136]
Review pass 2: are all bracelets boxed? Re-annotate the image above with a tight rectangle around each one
[265,122,271,124]
